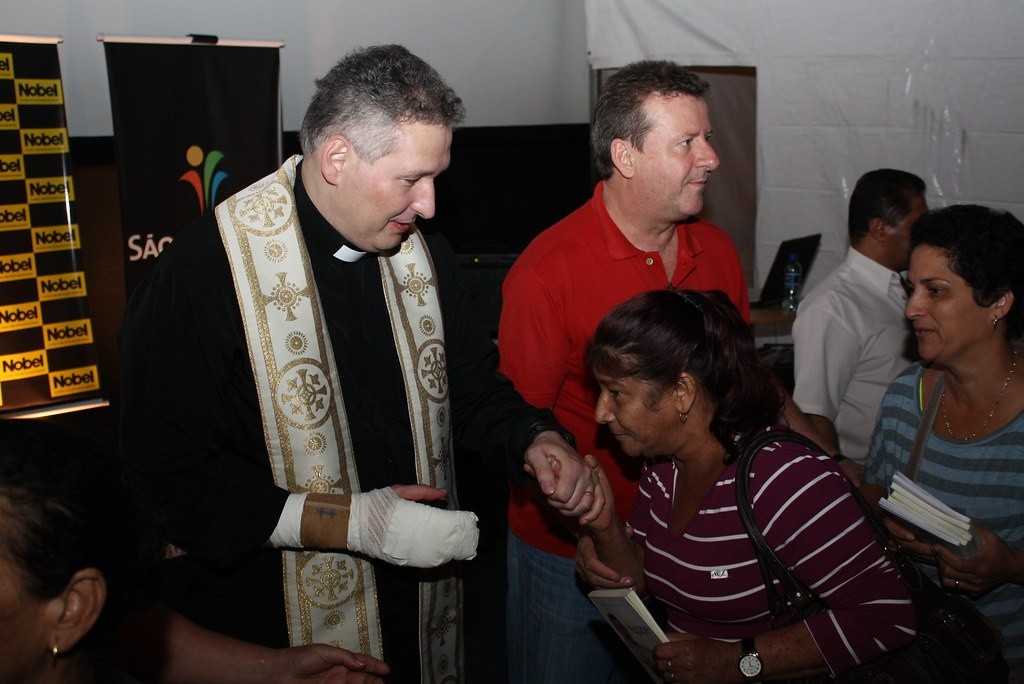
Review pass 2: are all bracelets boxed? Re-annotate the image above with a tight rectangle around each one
[833,454,847,462]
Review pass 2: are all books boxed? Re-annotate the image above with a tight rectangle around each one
[588,588,671,684]
[878,470,979,560]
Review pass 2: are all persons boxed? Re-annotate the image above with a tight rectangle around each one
[862,204,1024,684]
[525,289,920,684]
[497,60,867,684]
[792,169,929,468]
[0,426,388,684]
[608,613,663,684]
[115,46,598,684]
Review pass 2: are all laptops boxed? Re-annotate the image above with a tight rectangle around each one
[748,232,822,308]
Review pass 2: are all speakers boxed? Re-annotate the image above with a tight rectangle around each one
[457,254,525,340]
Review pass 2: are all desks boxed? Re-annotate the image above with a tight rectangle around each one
[751,308,797,349]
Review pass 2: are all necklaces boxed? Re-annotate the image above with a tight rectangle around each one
[941,346,1016,441]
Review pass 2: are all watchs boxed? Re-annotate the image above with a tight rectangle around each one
[529,421,577,450]
[738,637,764,682]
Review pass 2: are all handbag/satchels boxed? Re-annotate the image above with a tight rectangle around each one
[735,432,1009,684]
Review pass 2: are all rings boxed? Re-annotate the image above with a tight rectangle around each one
[955,580,959,587]
[585,492,594,497]
[664,660,671,672]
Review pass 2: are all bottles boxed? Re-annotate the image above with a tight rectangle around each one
[780,253,802,312]
